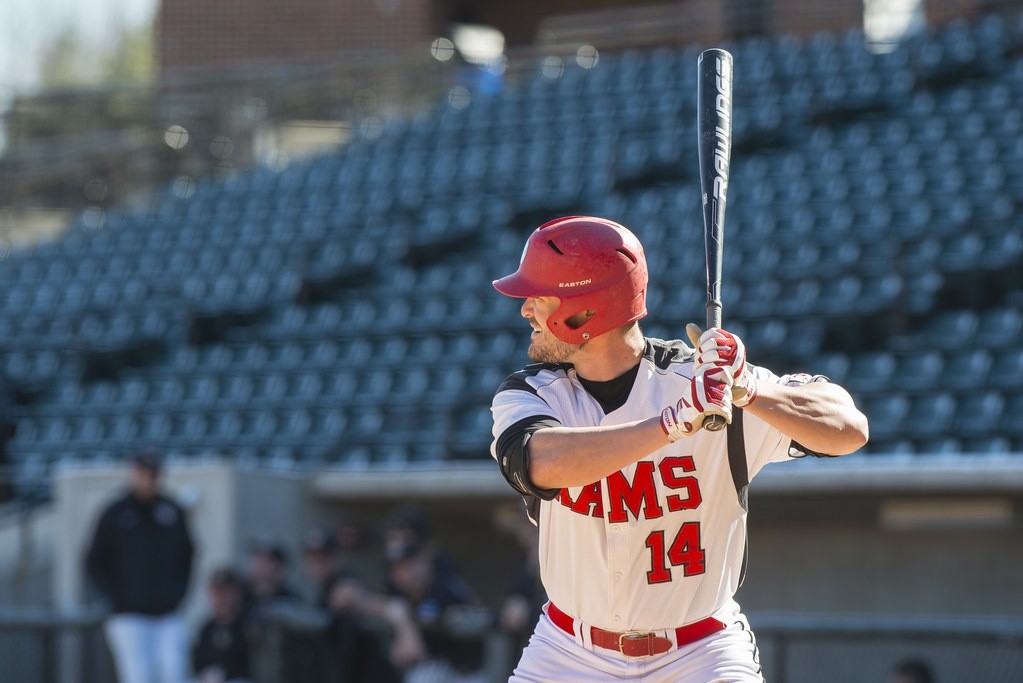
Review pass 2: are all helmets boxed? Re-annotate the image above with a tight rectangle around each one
[492,216,647,344]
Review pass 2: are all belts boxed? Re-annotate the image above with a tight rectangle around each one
[548,602,726,657]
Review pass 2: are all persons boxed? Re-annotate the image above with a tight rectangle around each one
[86,446,192,683]
[192,519,537,683]
[489,216,869,683]
[891,658,937,683]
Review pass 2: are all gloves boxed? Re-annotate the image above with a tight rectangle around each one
[686,323,758,408]
[660,363,732,443]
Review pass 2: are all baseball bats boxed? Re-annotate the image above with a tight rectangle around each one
[697,48,735,434]
